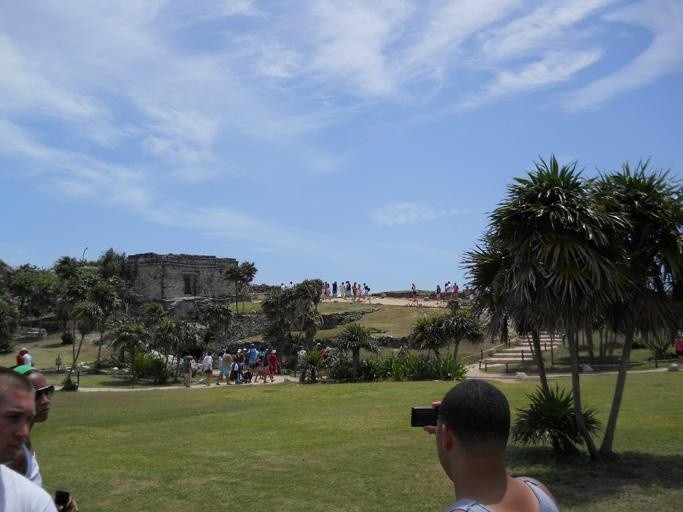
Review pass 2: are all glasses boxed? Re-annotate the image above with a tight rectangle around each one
[35,385,54,400]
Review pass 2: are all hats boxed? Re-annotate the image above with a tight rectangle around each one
[19,348,28,352]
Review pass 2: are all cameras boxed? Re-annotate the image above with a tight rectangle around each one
[411,407,438,427]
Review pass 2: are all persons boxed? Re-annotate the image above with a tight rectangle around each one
[321,281,372,302]
[411,283,417,302]
[464,286,479,300]
[436,281,459,303]
[297,345,307,368]
[424,378,562,512]
[0,347,80,512]
[403,346,410,357]
[280,283,285,294]
[316,343,334,359]
[289,281,293,288]
[675,337,683,363]
[181,343,280,388]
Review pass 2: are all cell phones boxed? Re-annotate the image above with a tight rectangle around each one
[55,491,69,512]
